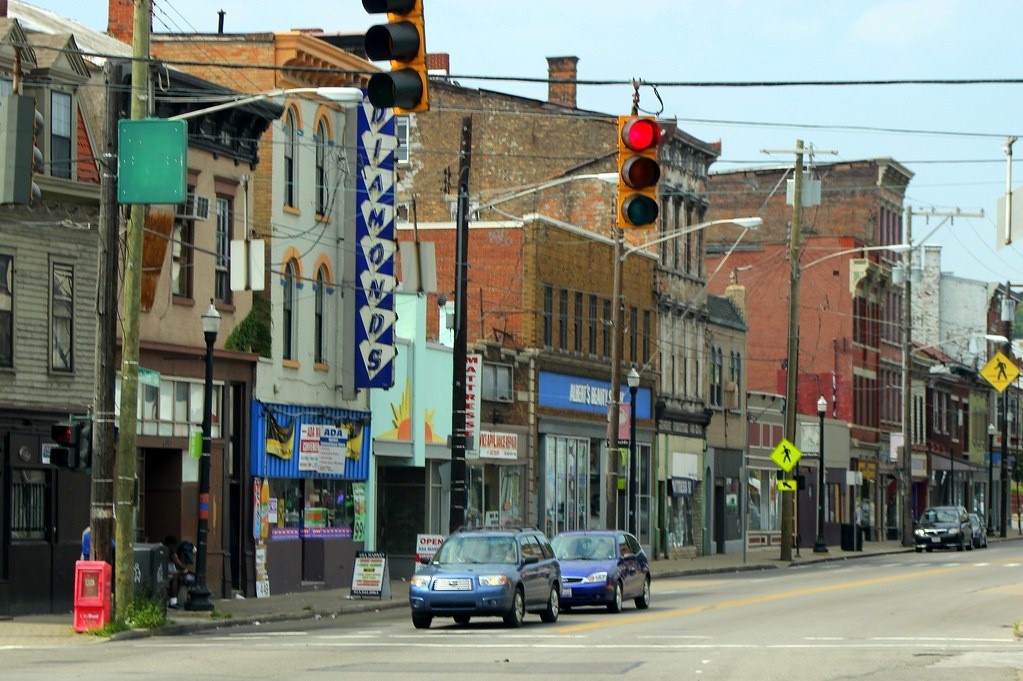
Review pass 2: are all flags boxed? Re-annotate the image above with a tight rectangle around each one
[800,422,820,453]
[890,433,905,461]
[607,403,630,438]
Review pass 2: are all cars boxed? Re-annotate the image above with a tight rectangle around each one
[913,506,973,553]
[550,530,651,613]
[968,513,987,549]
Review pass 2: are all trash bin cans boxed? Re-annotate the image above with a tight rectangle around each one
[840,523,863,551]
[133,541,170,627]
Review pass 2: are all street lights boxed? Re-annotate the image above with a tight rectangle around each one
[183,298,222,612]
[115,1,362,623]
[813,394,828,552]
[986,421,997,537]
[449,117,619,535]
[607,216,763,526]
[627,364,641,537]
[900,333,1009,548]
[780,243,910,561]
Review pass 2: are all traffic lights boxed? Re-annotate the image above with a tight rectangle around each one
[0,94,42,210]
[617,115,660,231]
[78,423,93,471]
[50,422,80,472]
[362,2,430,117]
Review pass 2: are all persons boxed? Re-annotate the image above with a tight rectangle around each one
[150,534,188,609]
[82,524,116,561]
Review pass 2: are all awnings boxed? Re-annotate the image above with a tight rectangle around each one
[930,450,989,471]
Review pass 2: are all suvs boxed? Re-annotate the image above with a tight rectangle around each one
[409,525,564,628]
